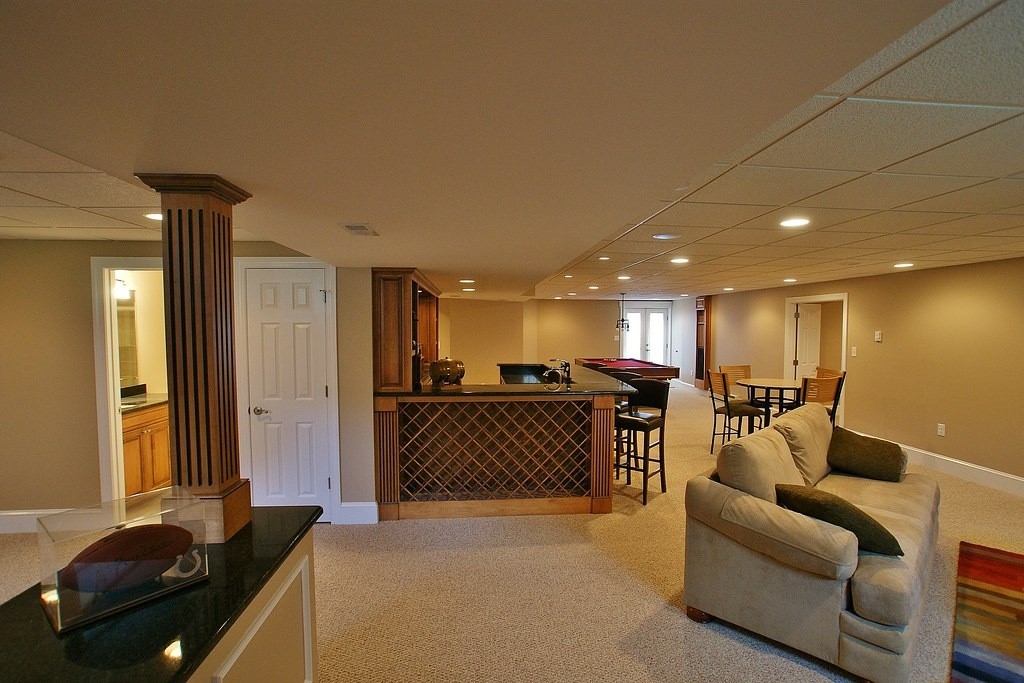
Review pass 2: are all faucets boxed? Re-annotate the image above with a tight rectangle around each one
[541,358,575,384]
[543,384,574,393]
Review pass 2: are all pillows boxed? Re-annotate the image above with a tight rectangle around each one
[827,424,903,483]
[773,481,906,557]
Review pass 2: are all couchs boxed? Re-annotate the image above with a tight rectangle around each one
[684,401,943,682]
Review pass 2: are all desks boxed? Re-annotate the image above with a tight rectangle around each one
[575,356,681,401]
[736,377,802,435]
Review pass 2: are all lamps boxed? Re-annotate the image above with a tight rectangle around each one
[616,291,629,331]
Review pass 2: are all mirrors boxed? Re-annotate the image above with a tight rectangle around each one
[117,288,138,390]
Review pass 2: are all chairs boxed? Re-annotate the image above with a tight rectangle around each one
[705,368,765,455]
[718,365,772,444]
[815,364,849,430]
[801,376,842,421]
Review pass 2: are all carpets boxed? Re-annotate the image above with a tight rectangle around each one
[950,539,1024,683]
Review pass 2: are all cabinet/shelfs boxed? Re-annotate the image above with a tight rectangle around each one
[371,266,438,392]
[122,402,171,511]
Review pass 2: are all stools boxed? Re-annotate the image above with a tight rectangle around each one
[582,361,670,505]
